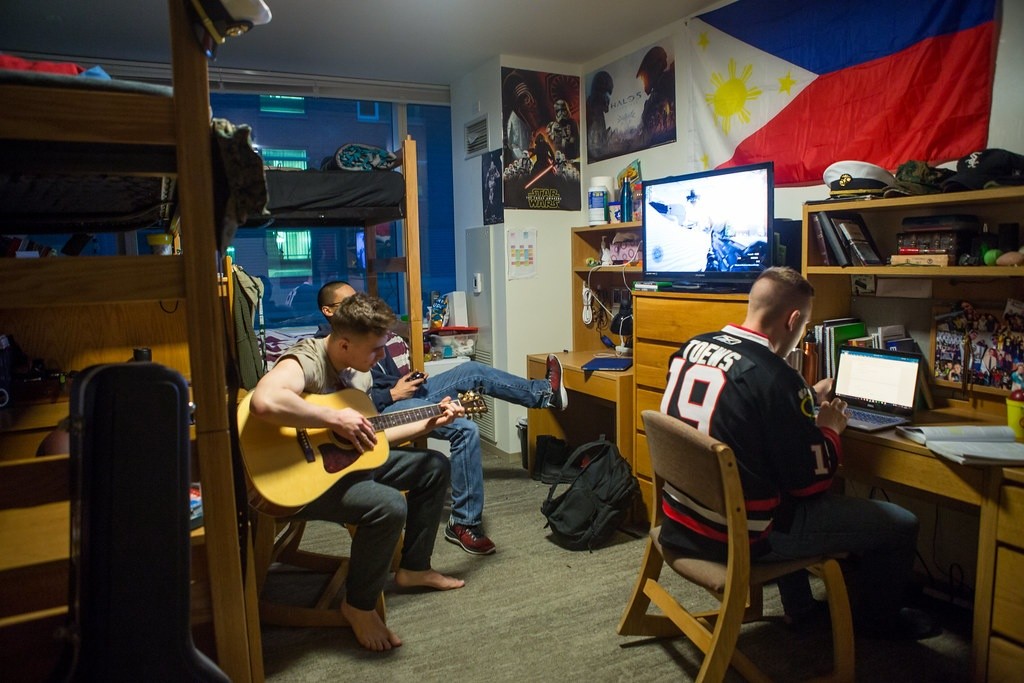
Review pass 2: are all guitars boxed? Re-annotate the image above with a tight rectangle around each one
[235,387,488,517]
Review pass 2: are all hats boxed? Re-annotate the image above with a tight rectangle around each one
[822,160,897,202]
[880,147,1024,199]
[190,0,273,61]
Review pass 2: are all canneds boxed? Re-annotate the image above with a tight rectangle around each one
[587,185,609,225]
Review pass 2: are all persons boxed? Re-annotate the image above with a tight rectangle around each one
[249,293,465,650]
[935,298,1023,390]
[313,281,569,556]
[659,266,920,672]
[242,269,320,321]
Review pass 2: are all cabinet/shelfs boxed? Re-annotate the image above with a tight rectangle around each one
[800,187,1024,683]
[621,290,752,528]
[527,221,644,475]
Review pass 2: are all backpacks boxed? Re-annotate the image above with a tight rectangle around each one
[541,433,640,550]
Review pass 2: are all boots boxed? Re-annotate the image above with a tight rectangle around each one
[531,434,559,481]
[540,439,583,485]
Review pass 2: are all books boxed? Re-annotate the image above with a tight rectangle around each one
[812,211,883,266]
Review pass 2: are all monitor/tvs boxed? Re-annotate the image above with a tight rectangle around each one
[641,161,776,291]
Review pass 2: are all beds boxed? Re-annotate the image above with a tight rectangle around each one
[0,1,423,683]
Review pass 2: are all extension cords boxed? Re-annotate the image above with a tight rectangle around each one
[616,345,633,352]
[923,587,972,608]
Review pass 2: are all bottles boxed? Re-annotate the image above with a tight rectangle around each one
[631,184,642,221]
[803,331,817,385]
[620,177,633,222]
[588,186,608,226]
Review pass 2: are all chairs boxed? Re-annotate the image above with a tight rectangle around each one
[236,384,408,630]
[616,410,856,683]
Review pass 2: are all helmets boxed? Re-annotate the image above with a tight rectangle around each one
[634,46,667,96]
[590,71,614,113]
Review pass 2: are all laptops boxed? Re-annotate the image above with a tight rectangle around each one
[581,357,634,371]
[812,344,924,431]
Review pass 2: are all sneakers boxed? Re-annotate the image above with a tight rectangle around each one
[443,520,497,555]
[545,353,568,412]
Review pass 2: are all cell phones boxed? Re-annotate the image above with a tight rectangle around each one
[404,372,424,381]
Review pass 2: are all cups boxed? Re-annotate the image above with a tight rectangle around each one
[1005,398,1024,442]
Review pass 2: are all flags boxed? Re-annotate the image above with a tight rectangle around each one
[687,1,1003,189]
